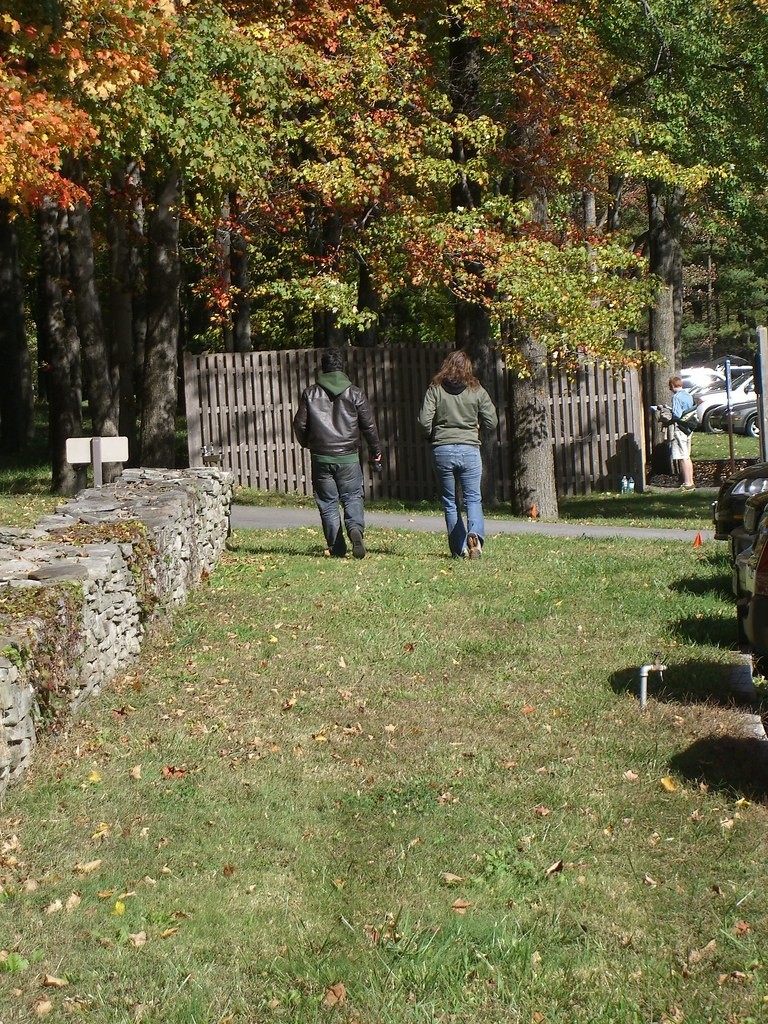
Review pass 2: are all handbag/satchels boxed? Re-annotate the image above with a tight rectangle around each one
[677,403,699,435]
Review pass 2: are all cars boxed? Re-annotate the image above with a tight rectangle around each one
[711,463,768,733]
[710,402,759,437]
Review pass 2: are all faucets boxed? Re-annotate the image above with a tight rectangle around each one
[650,651,670,671]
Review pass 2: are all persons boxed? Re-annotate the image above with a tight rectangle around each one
[655,375,698,492]
[292,348,381,558]
[418,347,498,559]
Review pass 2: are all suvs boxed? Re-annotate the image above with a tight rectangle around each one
[682,364,753,394]
[691,372,760,434]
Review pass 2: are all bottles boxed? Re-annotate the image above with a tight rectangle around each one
[628,477,635,494]
[621,475,628,494]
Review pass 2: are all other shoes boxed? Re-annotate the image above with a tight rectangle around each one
[350,528,365,560]
[324,549,334,558]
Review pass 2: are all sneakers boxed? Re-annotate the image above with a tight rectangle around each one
[461,548,469,557]
[467,533,482,559]
[670,483,695,493]
